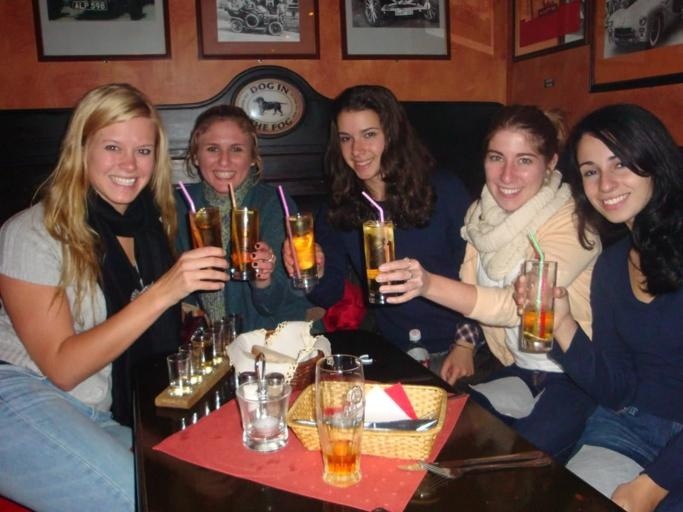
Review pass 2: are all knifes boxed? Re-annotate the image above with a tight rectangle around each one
[397,451,543,472]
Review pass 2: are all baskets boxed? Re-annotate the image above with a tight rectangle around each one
[284,379,449,463]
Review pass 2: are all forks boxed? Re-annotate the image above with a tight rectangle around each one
[417,458,550,479]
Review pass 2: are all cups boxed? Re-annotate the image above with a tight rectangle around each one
[288,213,319,289]
[362,220,397,306]
[315,354,366,488]
[167,312,236,398]
[188,207,226,293]
[231,207,260,281]
[518,260,557,354]
[235,378,292,453]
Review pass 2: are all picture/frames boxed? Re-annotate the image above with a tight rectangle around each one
[588,0,683,93]
[195,0,320,59]
[32,0,171,61]
[340,0,451,60]
[512,0,589,63]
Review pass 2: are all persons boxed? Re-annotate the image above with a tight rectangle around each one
[283,84,474,353]
[0,84,230,511]
[512,104,683,511]
[375,104,603,427]
[151,105,325,356]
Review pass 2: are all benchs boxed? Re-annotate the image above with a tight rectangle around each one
[0,65,503,222]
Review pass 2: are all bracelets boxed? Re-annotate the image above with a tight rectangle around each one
[453,343,475,355]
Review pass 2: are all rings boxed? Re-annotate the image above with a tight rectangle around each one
[404,268,415,281]
[265,252,274,264]
[404,255,413,268]
